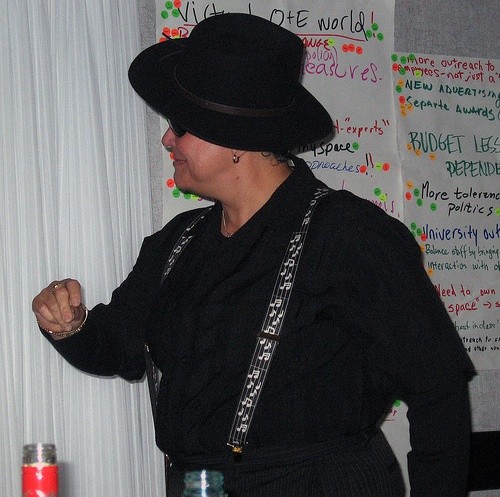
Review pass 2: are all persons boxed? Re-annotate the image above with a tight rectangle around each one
[31,13,478,497]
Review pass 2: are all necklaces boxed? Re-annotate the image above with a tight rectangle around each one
[219,209,236,239]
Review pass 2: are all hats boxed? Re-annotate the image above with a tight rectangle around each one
[128,13,334,151]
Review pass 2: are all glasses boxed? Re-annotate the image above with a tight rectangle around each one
[166,116,188,139]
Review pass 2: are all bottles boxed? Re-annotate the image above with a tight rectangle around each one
[185,471,224,497]
[21,443,60,497]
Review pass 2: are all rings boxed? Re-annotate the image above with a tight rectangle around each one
[51,283,65,292]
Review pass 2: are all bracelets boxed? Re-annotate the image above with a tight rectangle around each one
[36,303,89,336]
